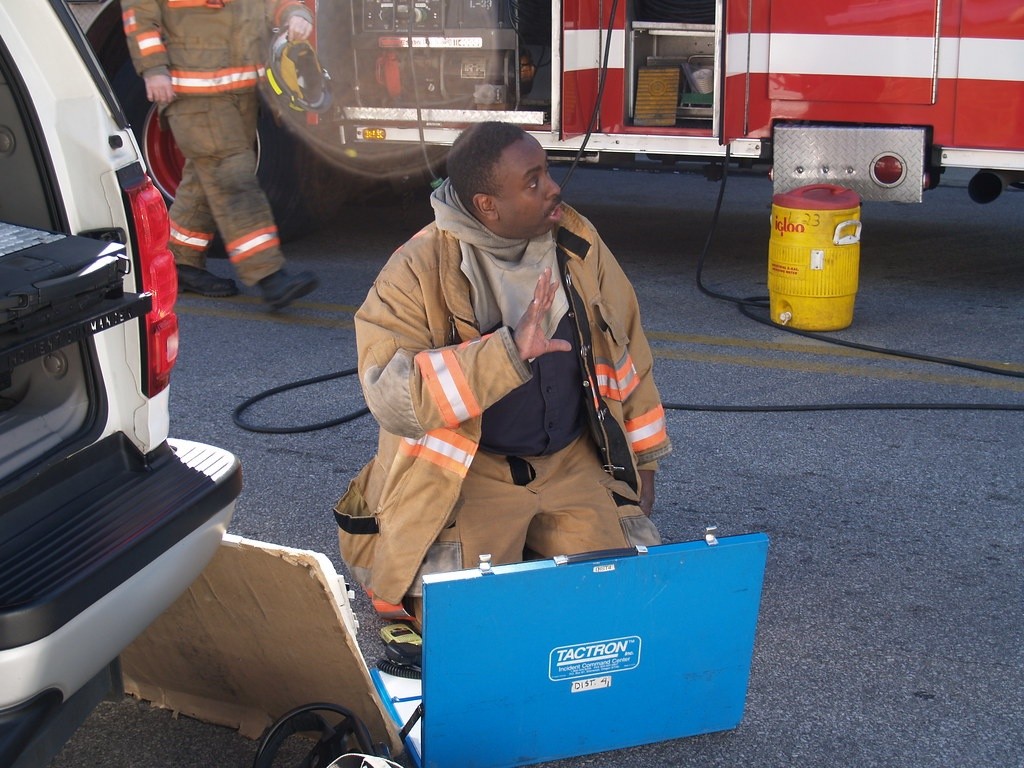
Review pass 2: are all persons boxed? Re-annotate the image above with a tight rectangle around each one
[334,121,673,634]
[122,0,322,314]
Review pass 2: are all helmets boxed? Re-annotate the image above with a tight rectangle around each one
[264,26,332,117]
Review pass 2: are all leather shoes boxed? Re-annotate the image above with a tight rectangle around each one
[259,266,321,311]
[176,264,240,297]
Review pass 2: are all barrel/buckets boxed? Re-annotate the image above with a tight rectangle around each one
[768,185,861,330]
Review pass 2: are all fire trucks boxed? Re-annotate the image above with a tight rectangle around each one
[76,0,1024,248]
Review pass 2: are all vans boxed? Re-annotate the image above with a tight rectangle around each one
[0,2,244,768]
